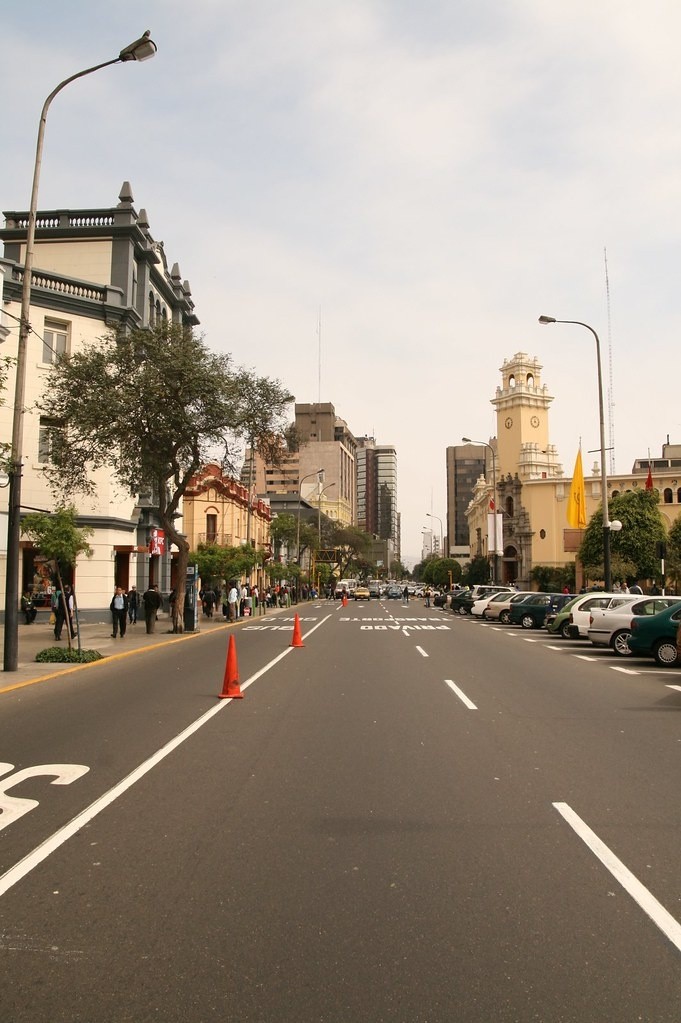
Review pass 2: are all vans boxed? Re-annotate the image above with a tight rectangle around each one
[471,585,520,601]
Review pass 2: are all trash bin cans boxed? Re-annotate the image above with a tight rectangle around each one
[245,597,252,608]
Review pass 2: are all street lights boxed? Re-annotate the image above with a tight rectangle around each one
[296,469,324,603]
[427,513,444,558]
[539,315,612,591]
[421,526,433,553]
[319,483,336,549]
[462,437,498,584]
[4,30,157,671]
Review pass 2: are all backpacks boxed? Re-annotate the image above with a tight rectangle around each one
[204,591,213,601]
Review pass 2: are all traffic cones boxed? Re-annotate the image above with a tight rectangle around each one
[288,612,306,648]
[342,595,348,607]
[218,634,244,699]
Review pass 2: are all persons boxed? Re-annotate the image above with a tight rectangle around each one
[592,583,598,590]
[51,583,77,641]
[263,583,318,608]
[563,586,569,594]
[110,585,141,638]
[21,590,37,625]
[613,576,644,595]
[426,587,430,608]
[452,584,470,592]
[199,584,259,623]
[651,584,660,594]
[30,592,33,602]
[143,584,176,634]
[341,586,348,600]
[329,586,335,600]
[579,585,586,594]
[404,586,408,603]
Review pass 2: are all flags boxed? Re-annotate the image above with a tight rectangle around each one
[490,498,495,510]
[567,450,586,528]
[646,467,653,491]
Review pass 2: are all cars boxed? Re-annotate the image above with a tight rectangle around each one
[627,601,681,667]
[471,592,516,620]
[509,593,570,628]
[450,590,474,614]
[587,596,681,657]
[484,592,544,623]
[544,591,609,638]
[335,579,440,601]
[567,594,650,638]
[434,590,463,610]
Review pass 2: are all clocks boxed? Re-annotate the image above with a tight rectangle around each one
[530,416,540,429]
[504,417,513,429]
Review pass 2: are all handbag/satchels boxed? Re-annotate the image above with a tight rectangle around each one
[48,611,56,625]
[314,594,318,598]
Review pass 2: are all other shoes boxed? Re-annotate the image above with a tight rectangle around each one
[71,632,78,639]
[110,634,116,638]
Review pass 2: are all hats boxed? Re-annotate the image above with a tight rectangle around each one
[149,585,155,589]
[132,585,136,588]
